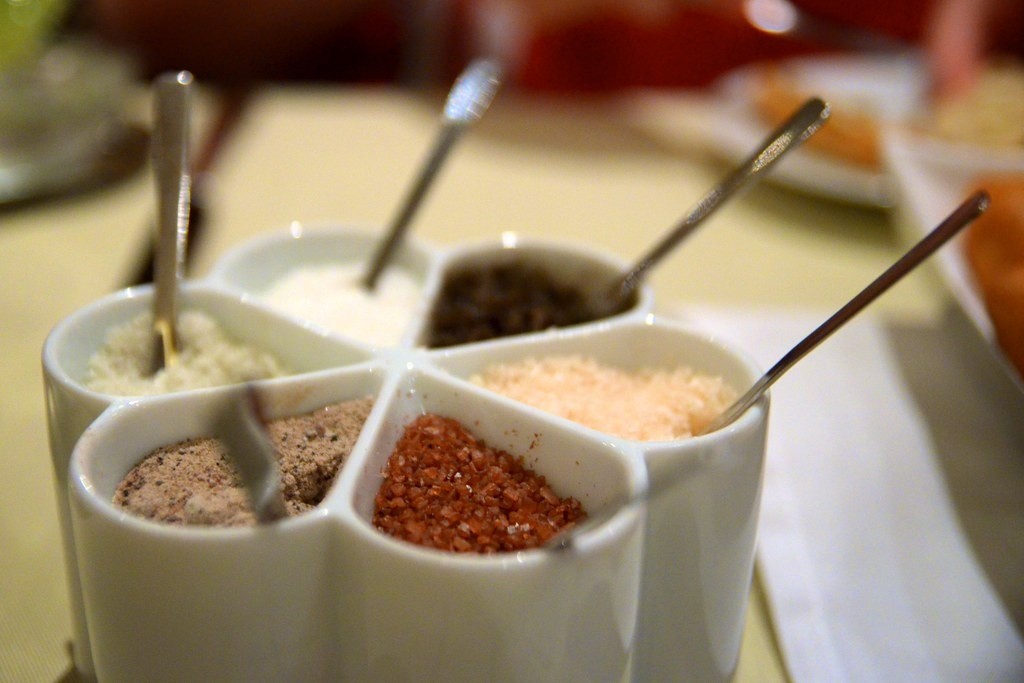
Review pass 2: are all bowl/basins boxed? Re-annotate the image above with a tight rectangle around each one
[42,225,769,682]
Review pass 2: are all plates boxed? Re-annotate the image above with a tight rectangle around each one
[640,66,1023,208]
[892,140,1024,394]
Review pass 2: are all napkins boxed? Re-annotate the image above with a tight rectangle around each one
[679,305,1024,683]
